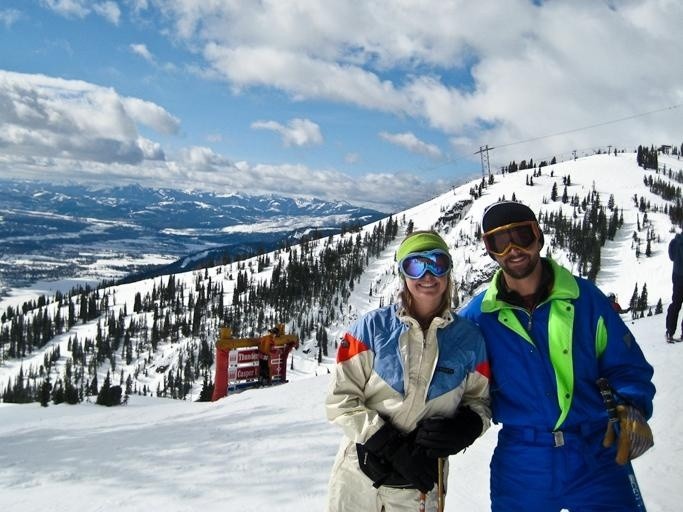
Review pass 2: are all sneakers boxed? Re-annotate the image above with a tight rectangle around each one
[666,330,675,343]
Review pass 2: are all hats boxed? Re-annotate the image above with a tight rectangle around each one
[396,231,449,264]
[482,200,544,261]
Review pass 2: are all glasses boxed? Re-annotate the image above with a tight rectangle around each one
[398,248,453,279]
[482,220,542,257]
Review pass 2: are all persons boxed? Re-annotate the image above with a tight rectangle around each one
[322,229,494,512]
[606,291,633,314]
[455,201,656,512]
[665,225,683,344]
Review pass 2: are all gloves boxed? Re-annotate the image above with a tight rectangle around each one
[602,404,655,466]
[420,408,483,462]
[362,421,442,495]
